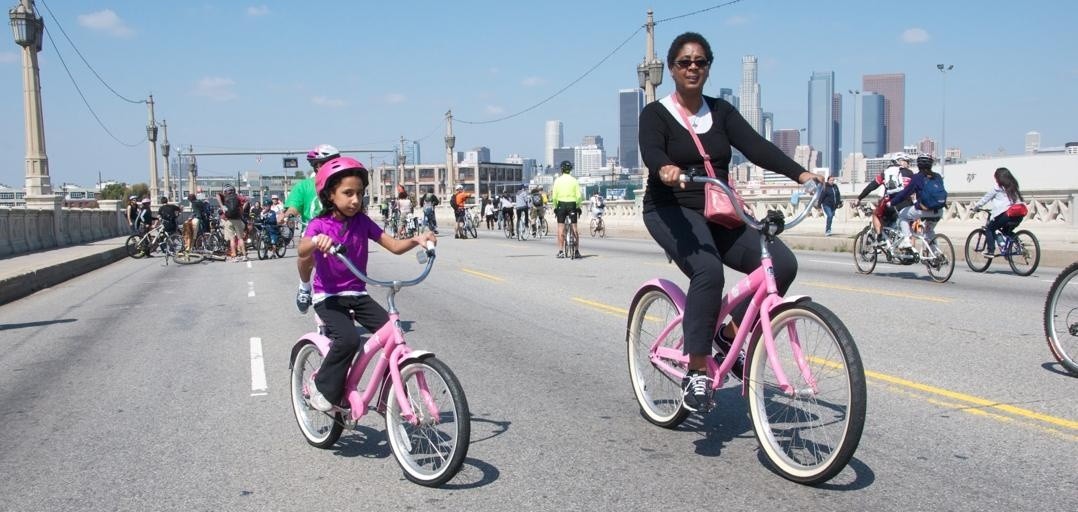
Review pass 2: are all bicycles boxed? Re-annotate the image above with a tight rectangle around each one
[1041,261,1077,380]
[859,207,917,266]
[625,159,868,487]
[848,197,955,283]
[288,234,470,488]
[554,208,581,260]
[588,209,605,238]
[963,205,1040,277]
[380,196,548,241]
[120,200,302,264]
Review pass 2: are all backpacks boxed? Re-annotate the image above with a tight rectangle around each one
[919,172,946,211]
[224,194,240,219]
[884,167,905,194]
[596,196,605,207]
[532,192,542,206]
[450,192,462,209]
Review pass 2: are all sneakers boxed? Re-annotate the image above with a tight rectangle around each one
[871,233,887,247]
[982,251,994,258]
[899,239,911,249]
[556,251,564,257]
[575,253,580,257]
[309,373,333,411]
[712,324,745,382]
[682,368,711,412]
[297,284,312,313]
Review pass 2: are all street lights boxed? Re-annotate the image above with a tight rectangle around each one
[443,111,457,197]
[396,136,408,196]
[935,63,954,178]
[141,94,162,208]
[798,128,807,169]
[848,89,859,191]
[159,121,174,202]
[367,152,376,207]
[635,9,665,189]
[7,0,64,202]
[187,146,198,200]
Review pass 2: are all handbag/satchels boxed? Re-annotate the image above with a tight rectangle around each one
[704,181,746,229]
[1007,204,1027,217]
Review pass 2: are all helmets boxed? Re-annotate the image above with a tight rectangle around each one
[223,184,235,195]
[130,195,150,204]
[456,185,463,190]
[890,152,933,167]
[262,199,273,206]
[560,161,572,171]
[307,146,368,199]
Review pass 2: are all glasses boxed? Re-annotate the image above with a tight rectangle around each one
[672,59,710,68]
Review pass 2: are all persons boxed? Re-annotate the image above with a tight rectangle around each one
[126,184,293,263]
[638,32,828,416]
[590,190,605,231]
[297,158,437,411]
[453,184,471,239]
[972,167,1024,258]
[853,151,945,266]
[818,176,841,236]
[379,185,440,236]
[282,145,342,311]
[481,183,548,236]
[552,161,582,259]
